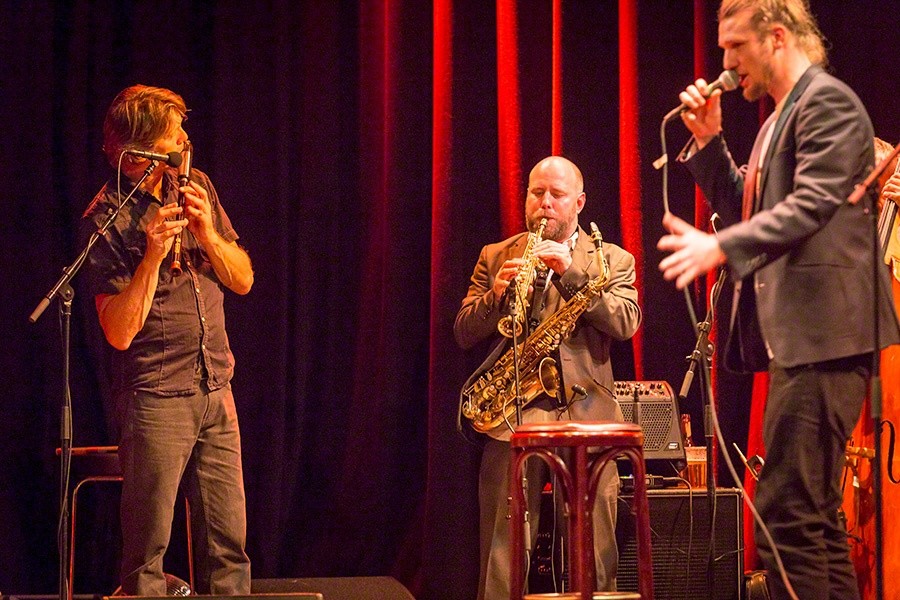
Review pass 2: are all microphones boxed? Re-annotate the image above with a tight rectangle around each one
[126,150,183,167]
[664,69,738,123]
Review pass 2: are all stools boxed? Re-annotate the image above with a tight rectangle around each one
[70,451,198,597]
[509,420,655,600]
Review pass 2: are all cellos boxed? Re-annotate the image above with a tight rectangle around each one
[837,149,900,600]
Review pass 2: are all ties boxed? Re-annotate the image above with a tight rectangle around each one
[741,108,778,221]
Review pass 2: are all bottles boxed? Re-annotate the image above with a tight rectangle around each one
[677,414,695,488]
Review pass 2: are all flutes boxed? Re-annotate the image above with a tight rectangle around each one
[497,218,550,337]
[169,140,190,276]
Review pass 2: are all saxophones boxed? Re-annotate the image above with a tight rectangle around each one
[462,222,611,433]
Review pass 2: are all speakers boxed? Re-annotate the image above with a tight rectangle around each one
[252,575,417,600]
[607,379,746,600]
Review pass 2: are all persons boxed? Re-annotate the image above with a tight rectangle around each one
[657,0,900,600]
[80,83,256,599]
[453,155,642,600]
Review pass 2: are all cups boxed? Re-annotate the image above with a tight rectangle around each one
[684,447,708,488]
